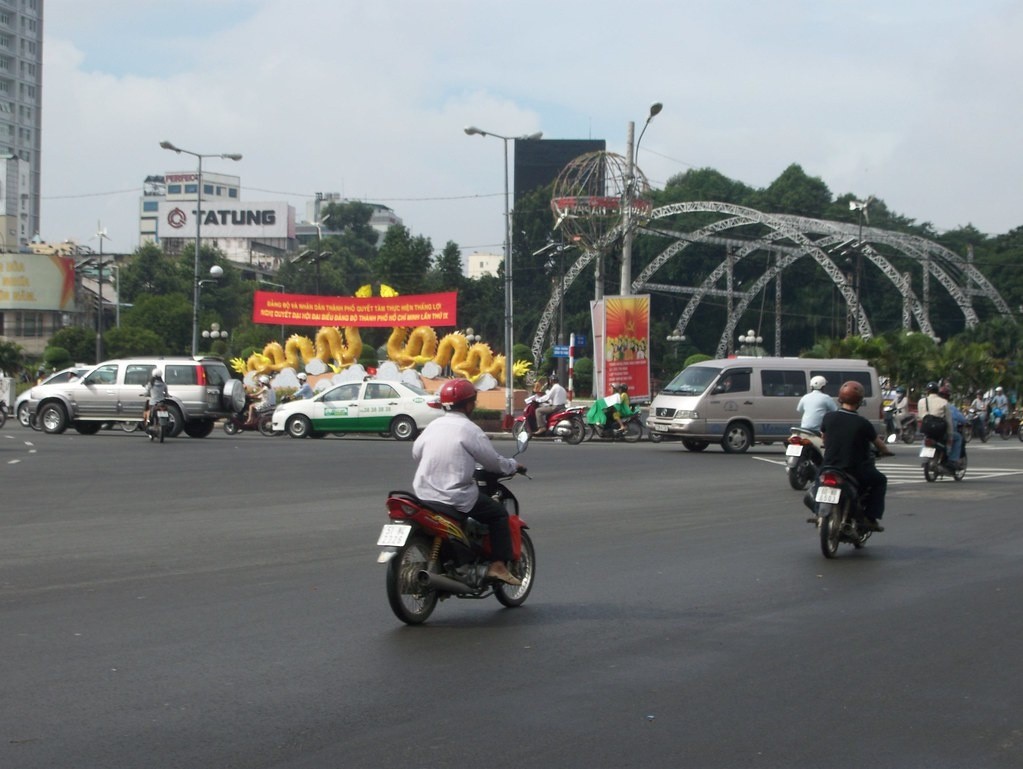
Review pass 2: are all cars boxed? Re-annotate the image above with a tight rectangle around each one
[271,375,446,441]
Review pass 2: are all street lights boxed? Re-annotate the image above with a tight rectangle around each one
[464,125,543,428]
[854,203,877,336]
[738,329,763,356]
[159,138,243,355]
[667,328,685,360]
[619,102,664,294]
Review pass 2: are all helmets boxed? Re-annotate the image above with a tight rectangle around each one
[924,382,939,392]
[611,382,620,389]
[837,381,866,404]
[975,389,983,394]
[809,375,828,390]
[995,386,1003,392]
[258,375,270,384]
[151,368,163,377]
[296,372,308,381]
[547,375,560,380]
[439,377,478,407]
[896,386,906,392]
[65,367,79,375]
[619,383,628,390]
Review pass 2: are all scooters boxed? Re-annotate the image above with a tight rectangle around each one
[223,392,350,438]
[139,393,172,443]
[580,398,647,443]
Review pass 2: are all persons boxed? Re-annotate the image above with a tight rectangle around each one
[143,369,169,425]
[244,376,276,424]
[796,376,838,431]
[600,383,630,437]
[37,371,46,386]
[411,379,522,586]
[532,375,566,434]
[68,369,79,383]
[815,381,889,532]
[918,382,966,469]
[291,372,313,399]
[890,386,908,435]
[971,386,1020,433]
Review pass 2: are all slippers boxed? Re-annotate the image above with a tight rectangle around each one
[487,570,522,585]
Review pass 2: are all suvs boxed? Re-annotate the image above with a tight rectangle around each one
[27,355,245,440]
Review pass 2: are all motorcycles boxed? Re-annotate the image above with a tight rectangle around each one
[784,426,897,561]
[376,431,537,624]
[883,396,1023,483]
[511,390,587,445]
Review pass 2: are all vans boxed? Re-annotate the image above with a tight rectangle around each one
[645,356,886,455]
[13,362,147,433]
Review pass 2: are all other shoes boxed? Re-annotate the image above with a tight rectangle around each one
[945,459,965,470]
[862,517,885,532]
[534,427,547,435]
[618,426,628,431]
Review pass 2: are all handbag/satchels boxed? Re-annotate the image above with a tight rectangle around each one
[919,414,948,440]
